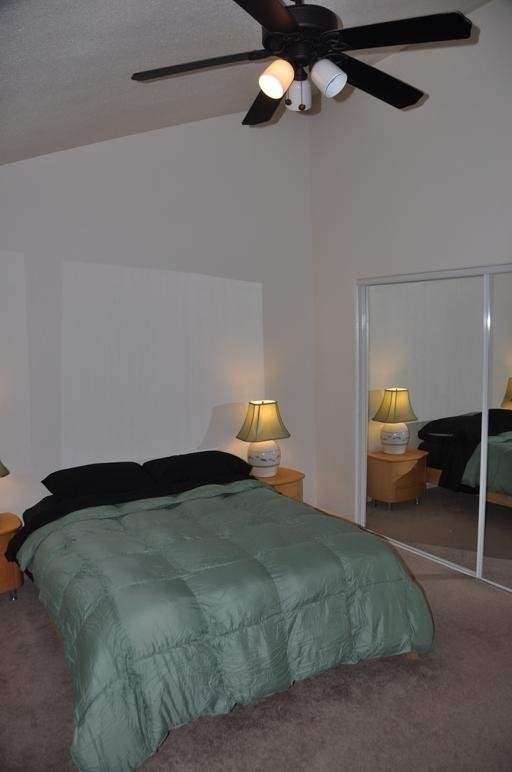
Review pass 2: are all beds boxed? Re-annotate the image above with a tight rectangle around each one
[23,452,434,772]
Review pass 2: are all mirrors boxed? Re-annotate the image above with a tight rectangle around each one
[350,265,510,596]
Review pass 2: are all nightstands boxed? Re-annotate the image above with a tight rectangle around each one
[258,466,304,502]
[1,512,25,601]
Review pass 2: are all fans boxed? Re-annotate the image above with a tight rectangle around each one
[128,0,472,128]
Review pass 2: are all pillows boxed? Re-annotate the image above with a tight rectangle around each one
[43,451,253,513]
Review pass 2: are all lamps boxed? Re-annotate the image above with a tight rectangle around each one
[237,400,291,478]
[258,57,349,112]
